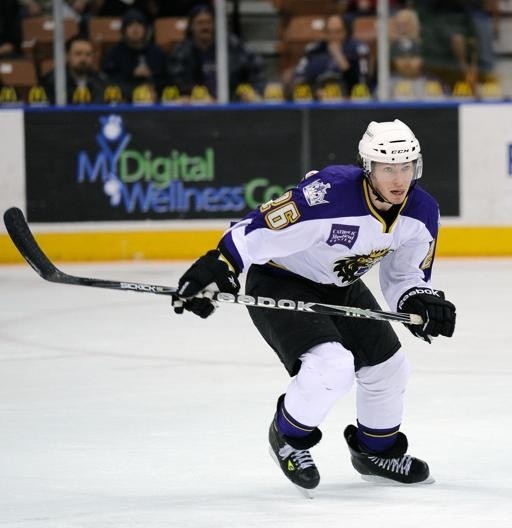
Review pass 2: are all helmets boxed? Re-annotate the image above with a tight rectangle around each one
[358,119,423,179]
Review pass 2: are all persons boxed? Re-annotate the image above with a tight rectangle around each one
[171,118,459,488]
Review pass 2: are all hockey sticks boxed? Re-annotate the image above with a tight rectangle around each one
[3,207,424,326]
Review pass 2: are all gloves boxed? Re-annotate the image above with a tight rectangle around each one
[171,250,240,318]
[397,286,456,344]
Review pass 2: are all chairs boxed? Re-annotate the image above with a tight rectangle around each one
[0,0,498,106]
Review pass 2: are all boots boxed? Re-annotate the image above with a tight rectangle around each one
[344,425,428,484]
[269,394,322,489]
